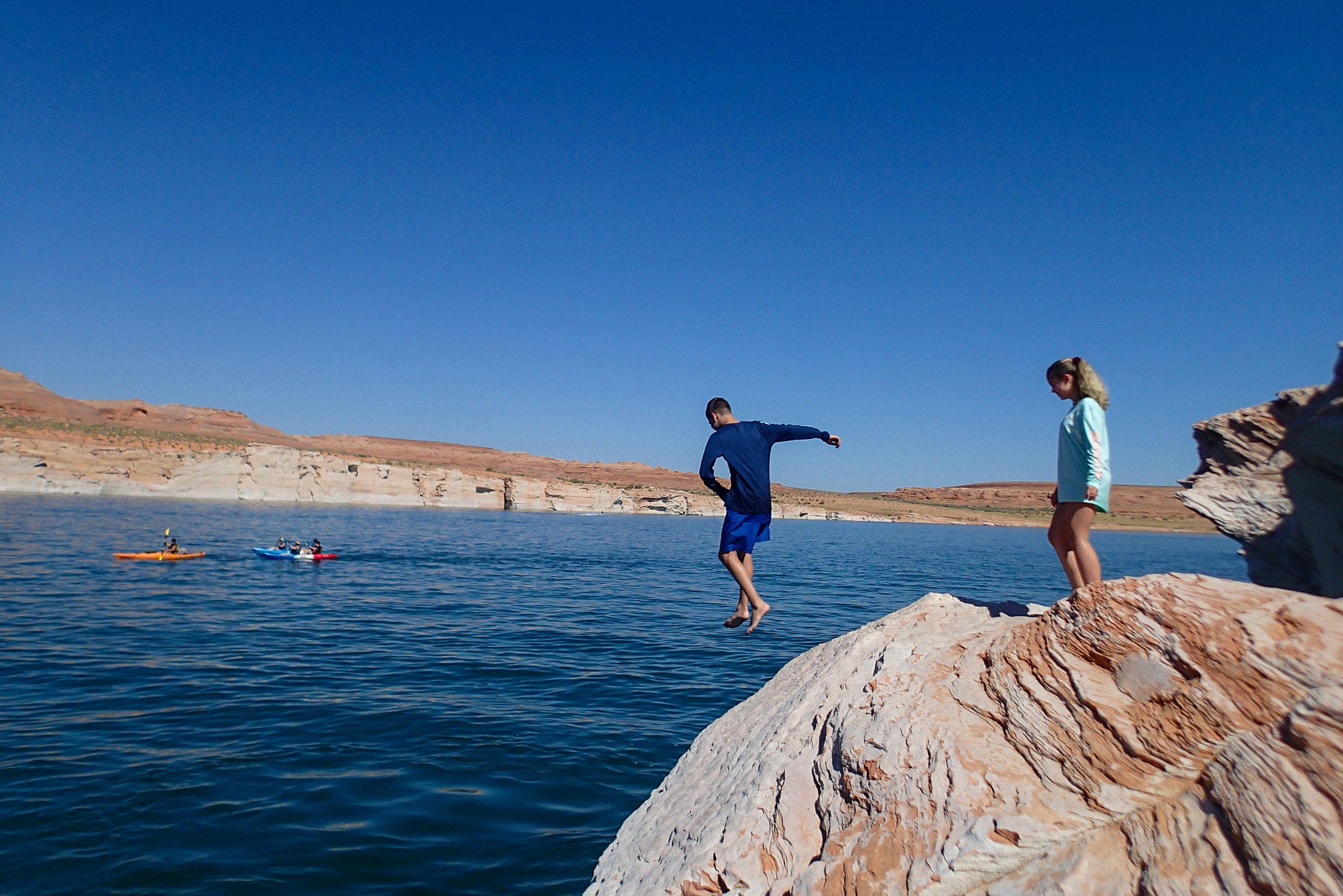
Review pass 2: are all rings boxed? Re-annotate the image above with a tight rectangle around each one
[1090,493,1095,494]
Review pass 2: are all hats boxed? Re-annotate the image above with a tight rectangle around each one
[280,538,284,541]
[313,538,317,542]
[314,541,320,544]
[171,538,176,542]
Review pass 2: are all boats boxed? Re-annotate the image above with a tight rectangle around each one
[111,547,207,561]
[250,546,339,560]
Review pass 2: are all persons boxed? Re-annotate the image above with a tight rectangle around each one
[1046,356,1111,590]
[162,538,177,553]
[268,538,322,555]
[699,397,841,634]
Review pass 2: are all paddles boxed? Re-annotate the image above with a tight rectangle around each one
[275,534,283,548]
[288,537,291,551]
[304,541,313,551]
[158,528,170,561]
